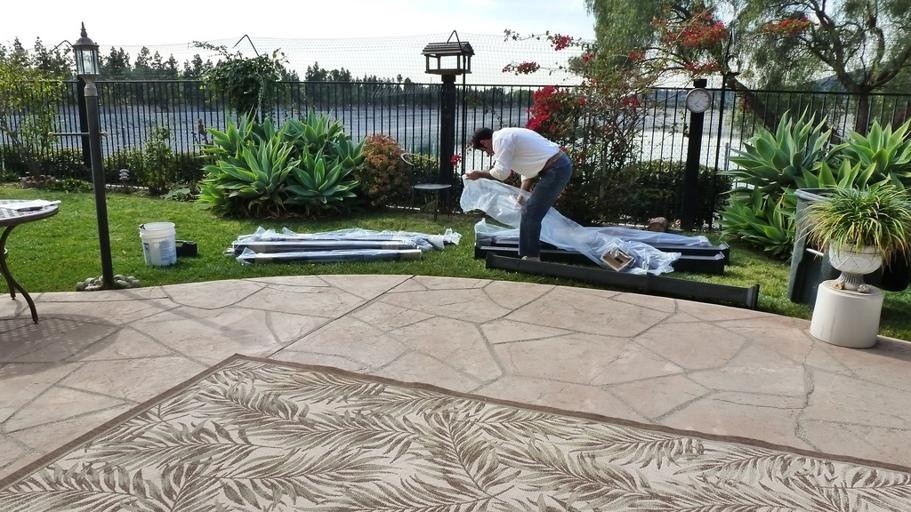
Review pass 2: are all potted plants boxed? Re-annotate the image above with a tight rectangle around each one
[794,184,911,294]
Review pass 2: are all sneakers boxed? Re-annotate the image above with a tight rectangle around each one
[521,256,542,262]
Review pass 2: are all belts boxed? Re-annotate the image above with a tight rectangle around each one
[542,149,565,169]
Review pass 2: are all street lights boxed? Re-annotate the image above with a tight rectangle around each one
[72,21,114,288]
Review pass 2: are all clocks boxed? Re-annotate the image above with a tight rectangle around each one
[685,87,710,113]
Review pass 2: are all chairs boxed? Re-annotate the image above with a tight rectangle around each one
[398,152,452,221]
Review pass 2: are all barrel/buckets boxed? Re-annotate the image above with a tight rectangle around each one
[138,221,177,267]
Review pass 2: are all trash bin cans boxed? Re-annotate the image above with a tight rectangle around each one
[789,186,842,304]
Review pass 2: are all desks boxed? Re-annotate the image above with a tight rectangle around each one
[0,199,59,324]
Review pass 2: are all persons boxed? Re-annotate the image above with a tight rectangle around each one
[466,127,572,263]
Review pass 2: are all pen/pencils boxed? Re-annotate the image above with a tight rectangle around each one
[17,207,43,211]
